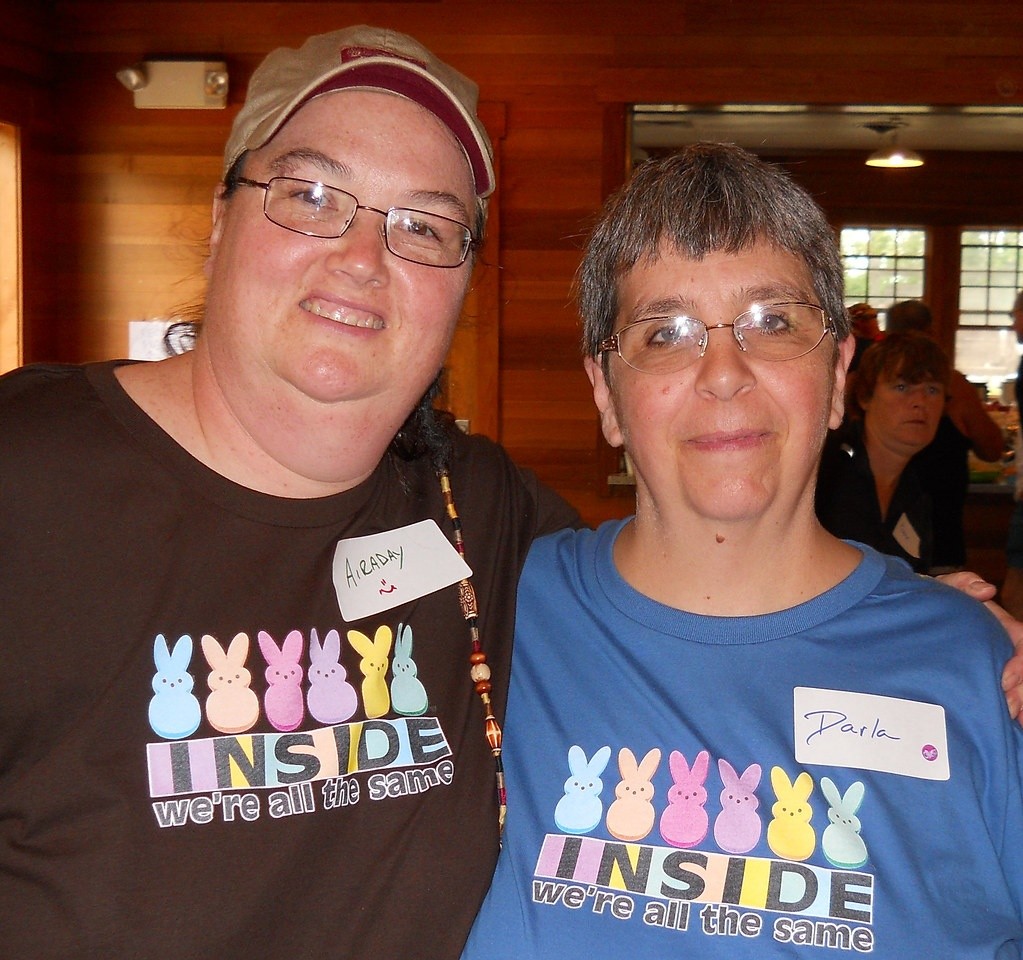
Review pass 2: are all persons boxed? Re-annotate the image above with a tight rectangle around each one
[0,23,1023,960]
[816,290,1023,574]
[458,143,1023,960]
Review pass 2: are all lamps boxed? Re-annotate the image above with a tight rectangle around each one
[118,59,229,110]
[865,116,924,168]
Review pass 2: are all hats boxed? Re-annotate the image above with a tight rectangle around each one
[219,23,497,218]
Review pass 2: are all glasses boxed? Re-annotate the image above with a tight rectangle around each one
[225,176,480,268]
[590,302,835,374]
